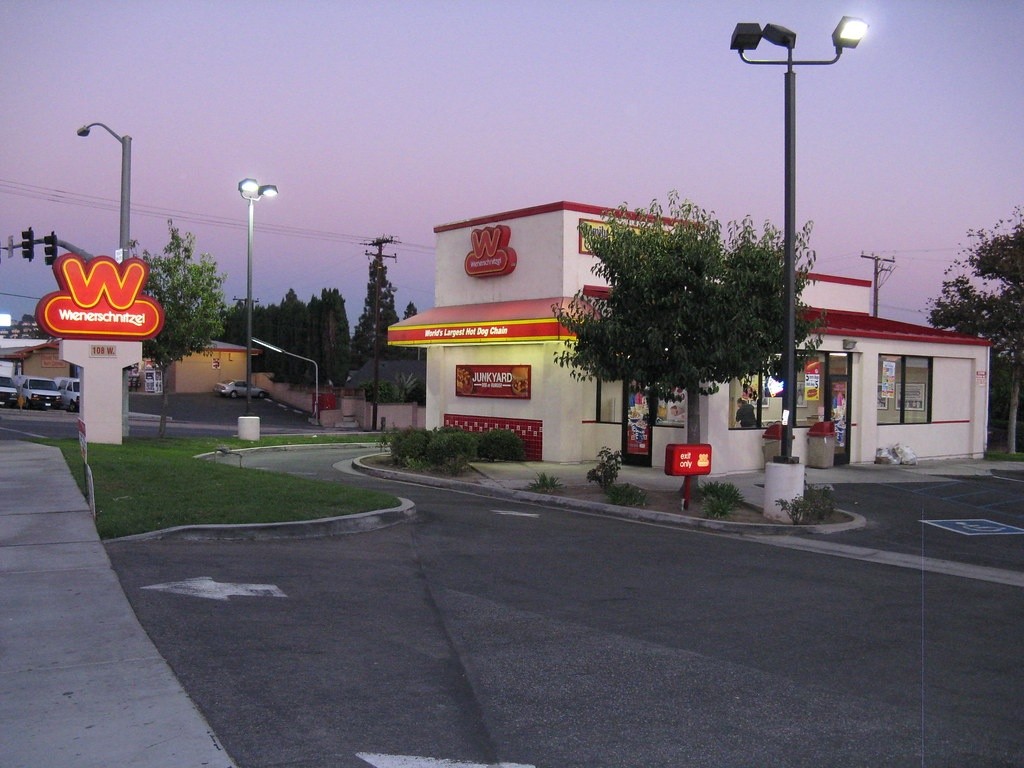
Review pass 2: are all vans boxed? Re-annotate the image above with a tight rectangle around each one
[54,376,80,412]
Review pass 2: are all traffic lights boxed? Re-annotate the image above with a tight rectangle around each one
[21,227,35,262]
[43,230,58,266]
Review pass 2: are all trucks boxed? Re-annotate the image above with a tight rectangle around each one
[0,374,17,408]
[12,375,62,411]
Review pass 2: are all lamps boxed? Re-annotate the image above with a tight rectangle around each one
[843,338,857,350]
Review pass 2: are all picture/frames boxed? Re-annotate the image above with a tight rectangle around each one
[747,397,771,409]
[796,380,808,408]
[894,382,926,412]
[876,382,889,411]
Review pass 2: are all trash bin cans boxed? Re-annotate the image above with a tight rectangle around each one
[806,421,838,470]
[761,424,796,473]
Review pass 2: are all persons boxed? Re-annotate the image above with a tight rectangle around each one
[735,398,757,427]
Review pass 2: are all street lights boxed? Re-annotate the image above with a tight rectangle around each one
[237,178,279,416]
[728,14,873,464]
[251,337,319,425]
[77,122,133,437]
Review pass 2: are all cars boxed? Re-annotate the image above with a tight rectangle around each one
[212,379,270,399]
